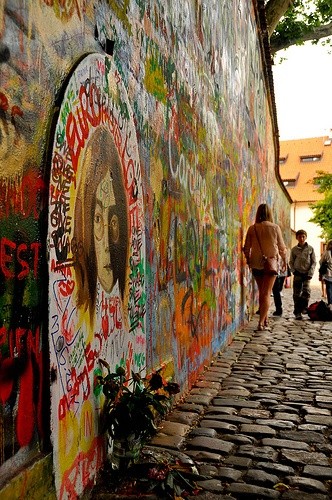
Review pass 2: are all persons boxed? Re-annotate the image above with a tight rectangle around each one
[316,237,332,317]
[255,246,286,318]
[242,203,289,333]
[287,228,317,322]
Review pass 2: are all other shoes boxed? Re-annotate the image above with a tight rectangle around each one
[303,308,308,313]
[256,310,260,314]
[273,311,282,316]
[259,322,268,330]
[295,313,302,319]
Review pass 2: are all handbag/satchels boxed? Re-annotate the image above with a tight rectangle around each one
[262,257,277,274]
[306,301,331,320]
